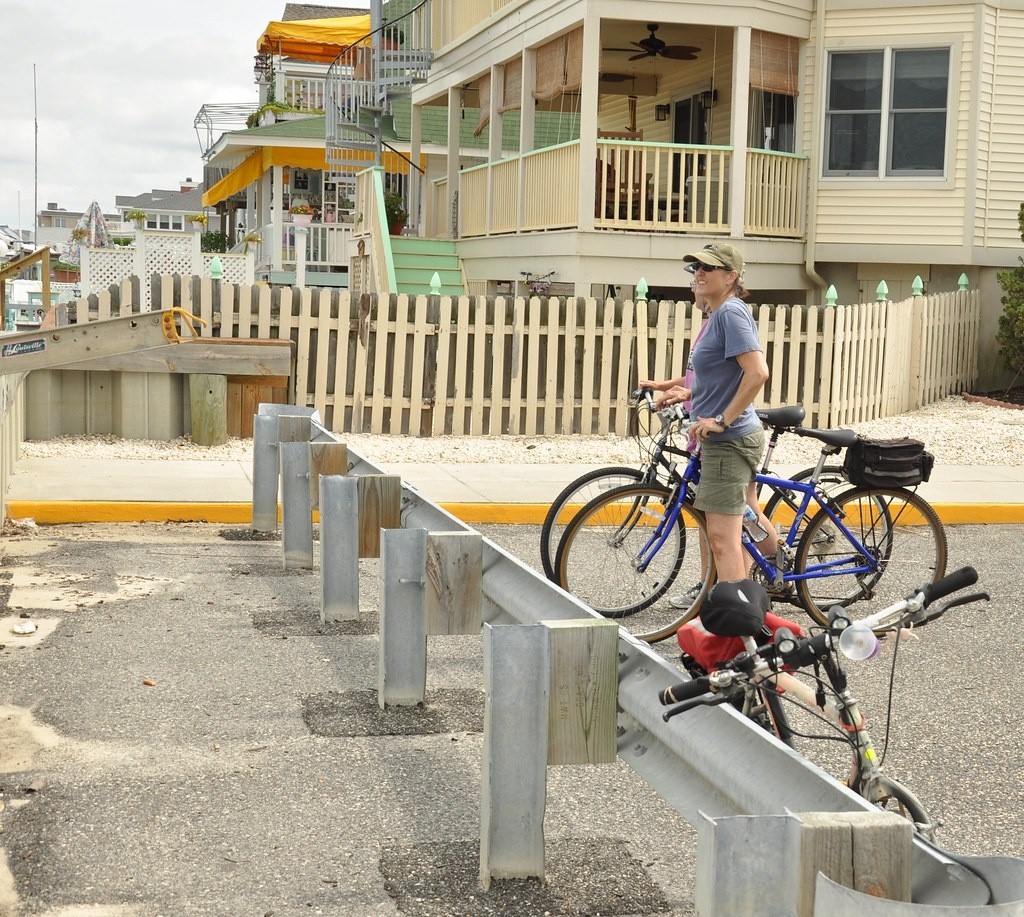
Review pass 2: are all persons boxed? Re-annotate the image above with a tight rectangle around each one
[640,272,755,609]
[655,242,797,596]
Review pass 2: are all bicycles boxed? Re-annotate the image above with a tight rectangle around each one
[657,563,992,849]
[539,386,896,621]
[552,401,950,647]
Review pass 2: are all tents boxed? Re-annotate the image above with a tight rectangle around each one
[256,12,399,71]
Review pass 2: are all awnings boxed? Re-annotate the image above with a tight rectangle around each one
[201,149,263,207]
[261,146,426,176]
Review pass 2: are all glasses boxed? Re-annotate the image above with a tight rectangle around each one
[691,262,732,273]
[689,280,696,286]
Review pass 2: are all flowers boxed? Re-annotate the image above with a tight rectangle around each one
[185,214,208,227]
[71,227,91,240]
[125,208,149,219]
[288,204,315,216]
[242,232,264,241]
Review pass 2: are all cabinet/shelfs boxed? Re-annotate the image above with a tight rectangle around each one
[289,168,313,194]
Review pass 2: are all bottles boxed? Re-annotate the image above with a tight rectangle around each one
[686,438,703,461]
[742,505,768,542]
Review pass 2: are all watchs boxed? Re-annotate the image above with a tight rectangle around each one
[716,415,729,430]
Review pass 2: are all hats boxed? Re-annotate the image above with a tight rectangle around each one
[683,241,744,274]
[684,263,696,274]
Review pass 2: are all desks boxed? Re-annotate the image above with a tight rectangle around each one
[648,191,688,233]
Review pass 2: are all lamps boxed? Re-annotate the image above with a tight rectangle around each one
[655,103,670,121]
[701,89,717,110]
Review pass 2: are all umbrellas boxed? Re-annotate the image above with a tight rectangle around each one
[59,200,116,284]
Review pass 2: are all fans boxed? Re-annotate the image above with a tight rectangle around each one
[602,24,702,61]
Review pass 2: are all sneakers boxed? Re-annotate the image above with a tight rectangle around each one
[668,580,704,610]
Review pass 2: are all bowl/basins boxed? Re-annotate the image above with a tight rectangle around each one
[342,214,354,223]
[344,202,354,208]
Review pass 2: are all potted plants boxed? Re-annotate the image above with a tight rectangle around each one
[382,26,407,50]
[383,191,409,235]
[50,263,80,283]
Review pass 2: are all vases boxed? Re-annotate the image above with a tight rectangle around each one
[249,241,257,249]
[193,221,202,230]
[131,219,144,230]
[80,236,87,243]
[294,214,311,226]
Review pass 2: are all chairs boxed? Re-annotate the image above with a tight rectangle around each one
[595,128,653,231]
